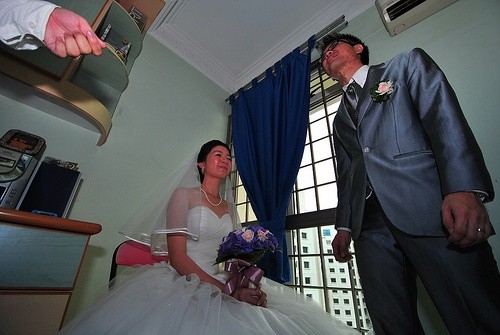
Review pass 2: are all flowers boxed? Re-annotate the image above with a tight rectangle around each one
[370,79,394,102]
[212,225,279,300]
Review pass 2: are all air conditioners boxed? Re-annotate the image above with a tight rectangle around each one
[374,0,462,37]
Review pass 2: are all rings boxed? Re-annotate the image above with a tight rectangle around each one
[477,228,486,233]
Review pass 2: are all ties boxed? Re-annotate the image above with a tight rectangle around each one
[346,82,361,109]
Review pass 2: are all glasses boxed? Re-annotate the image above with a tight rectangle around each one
[319,38,353,63]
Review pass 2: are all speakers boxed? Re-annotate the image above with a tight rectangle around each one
[15,160,83,219]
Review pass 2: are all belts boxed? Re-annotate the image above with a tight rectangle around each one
[364,183,374,199]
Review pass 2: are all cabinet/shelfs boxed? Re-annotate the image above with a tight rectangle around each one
[0,0,186,145]
[0,207,104,335]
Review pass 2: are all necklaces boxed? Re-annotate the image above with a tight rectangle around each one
[200,184,222,206]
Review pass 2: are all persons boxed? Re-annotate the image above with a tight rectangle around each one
[0,0,107,58]
[58,140,363,335]
[316,33,500,335]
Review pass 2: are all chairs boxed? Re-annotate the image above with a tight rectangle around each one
[109,240,170,291]
